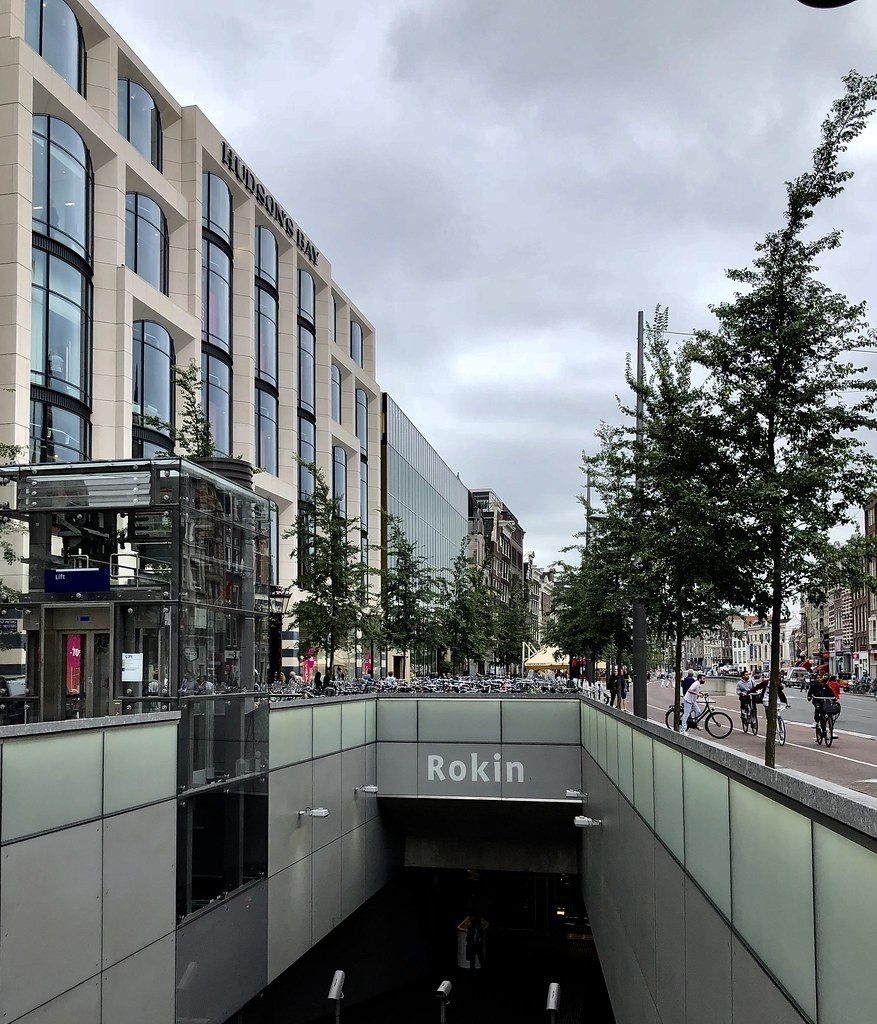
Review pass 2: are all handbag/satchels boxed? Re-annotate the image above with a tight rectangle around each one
[821,701,841,714]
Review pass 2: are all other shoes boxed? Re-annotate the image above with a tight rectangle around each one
[811,722,819,728]
[683,731,689,735]
[786,704,791,708]
[829,732,839,737]
[697,725,703,730]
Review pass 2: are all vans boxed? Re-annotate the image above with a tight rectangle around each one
[781,667,809,688]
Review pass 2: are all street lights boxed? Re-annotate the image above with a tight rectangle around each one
[799,612,808,659]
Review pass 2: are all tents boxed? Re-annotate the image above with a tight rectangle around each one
[525,648,618,671]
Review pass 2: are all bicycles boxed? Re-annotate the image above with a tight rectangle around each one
[775,705,791,746]
[811,694,839,747]
[740,698,763,735]
[664,693,734,739]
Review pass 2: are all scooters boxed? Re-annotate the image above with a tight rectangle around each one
[842,681,871,694]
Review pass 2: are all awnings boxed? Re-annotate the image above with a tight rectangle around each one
[799,661,812,669]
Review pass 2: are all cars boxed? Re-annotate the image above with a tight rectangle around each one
[716,666,739,676]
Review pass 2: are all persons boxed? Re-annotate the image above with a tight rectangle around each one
[800,674,807,692]
[680,673,709,735]
[314,672,322,689]
[681,669,695,696]
[384,672,395,685]
[466,916,484,972]
[164,674,214,695]
[363,669,371,681]
[555,669,567,679]
[149,671,158,708]
[736,671,757,721]
[861,671,870,684]
[746,672,787,719]
[323,668,336,690]
[288,671,304,686]
[274,672,286,683]
[336,668,344,681]
[608,667,675,711]
[807,674,841,738]
